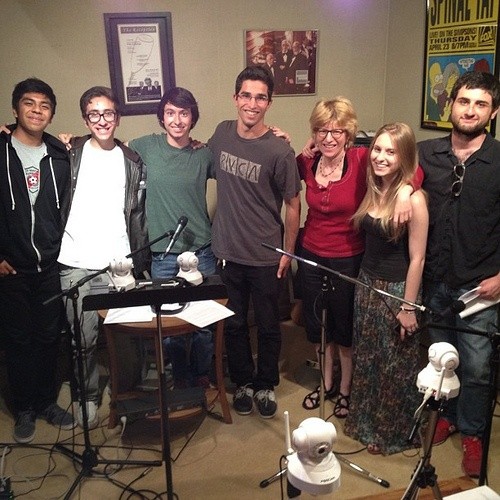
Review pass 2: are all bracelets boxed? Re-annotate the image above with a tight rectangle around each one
[400,306,416,312]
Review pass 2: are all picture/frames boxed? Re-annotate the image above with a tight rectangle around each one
[99,11,178,117]
[420,0,500,132]
[242,28,320,96]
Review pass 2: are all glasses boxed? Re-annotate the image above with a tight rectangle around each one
[85,111,118,123]
[451,164,465,196]
[317,128,345,139]
[237,93,272,103]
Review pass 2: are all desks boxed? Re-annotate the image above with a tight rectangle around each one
[96,298,234,429]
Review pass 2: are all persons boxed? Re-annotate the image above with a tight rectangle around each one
[59,86,218,393]
[135,79,161,95]
[342,121,430,455]
[0,78,207,444]
[270,95,425,419]
[204,65,307,417]
[261,37,316,93]
[301,70,500,479]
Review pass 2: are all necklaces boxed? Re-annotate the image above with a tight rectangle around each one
[319,155,344,177]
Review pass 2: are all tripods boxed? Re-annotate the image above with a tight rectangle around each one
[42,230,162,500]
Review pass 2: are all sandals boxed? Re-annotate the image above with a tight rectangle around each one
[334,392,351,418]
[303,385,337,410]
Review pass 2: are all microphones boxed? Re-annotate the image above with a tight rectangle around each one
[159,216,188,260]
[416,299,466,333]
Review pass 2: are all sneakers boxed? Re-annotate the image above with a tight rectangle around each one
[234,383,254,414]
[462,436,483,478]
[77,401,99,430]
[432,416,456,446]
[254,389,278,419]
[14,411,37,443]
[36,404,79,431]
